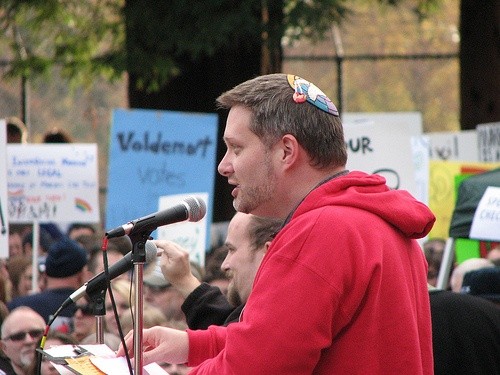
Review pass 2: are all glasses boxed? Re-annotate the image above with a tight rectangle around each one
[1,328,45,342]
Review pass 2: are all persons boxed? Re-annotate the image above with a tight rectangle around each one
[424,238,500,375]
[117,74,436,375]
[0,122,284,375]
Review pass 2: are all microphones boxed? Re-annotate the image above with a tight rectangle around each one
[104,194,206,239]
[61,240,157,308]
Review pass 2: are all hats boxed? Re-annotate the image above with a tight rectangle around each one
[45,240,89,278]
